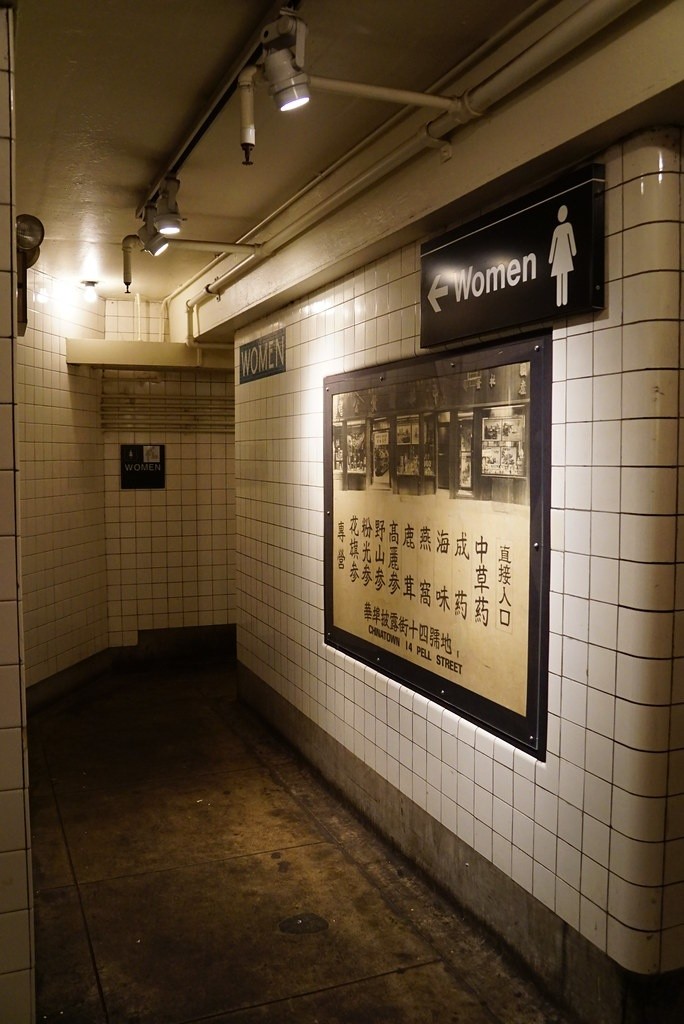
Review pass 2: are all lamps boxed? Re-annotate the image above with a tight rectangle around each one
[153,196,181,235]
[136,223,170,257]
[264,46,311,114]
[16,213,47,252]
[81,280,98,303]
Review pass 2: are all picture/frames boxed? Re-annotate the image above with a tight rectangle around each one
[320,331,552,768]
